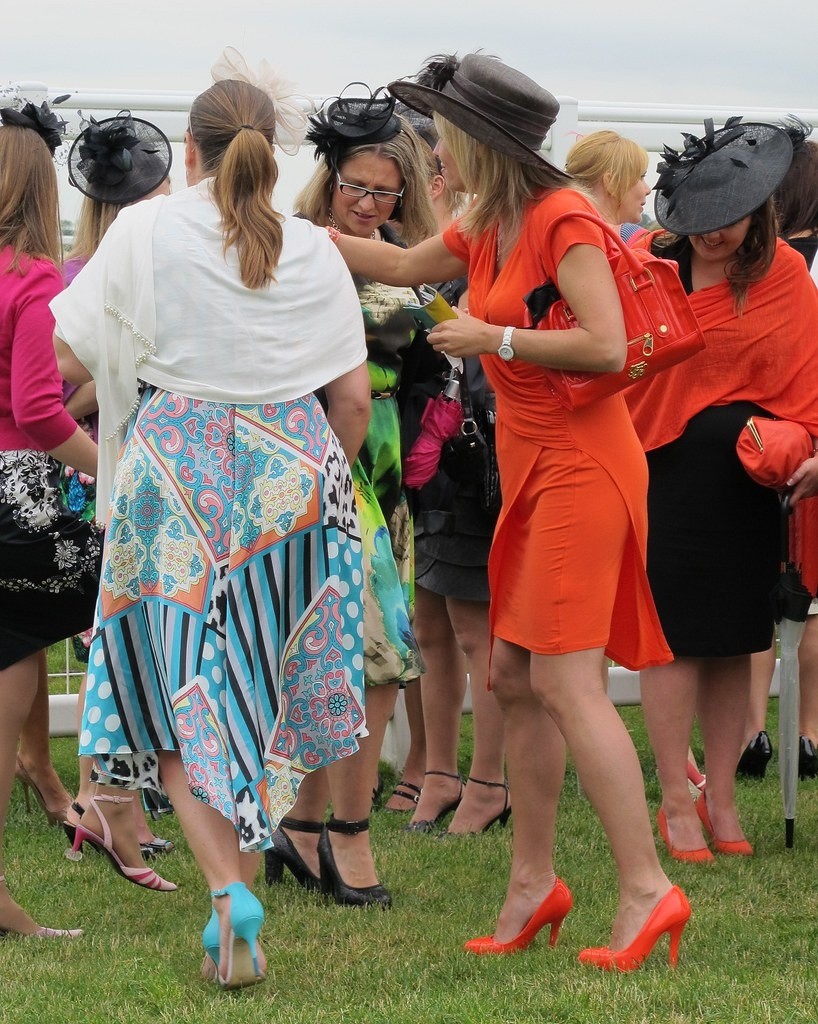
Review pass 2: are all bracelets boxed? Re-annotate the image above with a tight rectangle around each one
[325,224,340,245]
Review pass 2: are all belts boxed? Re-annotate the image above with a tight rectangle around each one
[371,385,399,400]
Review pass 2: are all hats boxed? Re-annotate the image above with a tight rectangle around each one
[388,54,577,181]
[654,114,793,236]
[67,106,173,204]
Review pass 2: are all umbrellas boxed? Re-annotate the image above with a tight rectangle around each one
[402,379,466,490]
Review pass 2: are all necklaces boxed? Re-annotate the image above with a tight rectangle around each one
[328,205,375,243]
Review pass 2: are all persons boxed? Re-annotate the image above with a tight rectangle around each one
[46,79,371,991]
[262,82,440,916]
[736,126,817,789]
[0,100,97,940]
[561,129,649,246]
[52,112,172,452]
[386,52,515,843]
[317,48,691,977]
[621,116,817,867]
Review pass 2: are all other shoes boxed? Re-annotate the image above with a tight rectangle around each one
[382,780,422,815]
[369,775,385,812]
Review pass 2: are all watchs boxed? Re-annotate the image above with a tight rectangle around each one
[498,326,516,363]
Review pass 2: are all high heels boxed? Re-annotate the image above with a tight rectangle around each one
[436,774,512,839]
[317,812,392,910]
[16,751,77,827]
[264,815,323,894]
[463,877,573,952]
[1,875,85,941]
[62,793,178,891]
[404,770,468,835]
[63,800,176,862]
[657,806,716,863]
[799,734,818,781]
[200,881,268,989]
[698,788,754,857]
[740,729,773,779]
[578,885,691,970]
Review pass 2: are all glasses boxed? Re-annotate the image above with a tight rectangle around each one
[334,167,407,207]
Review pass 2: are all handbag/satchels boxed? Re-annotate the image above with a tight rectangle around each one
[400,328,499,523]
[525,208,709,412]
[735,414,817,498]
